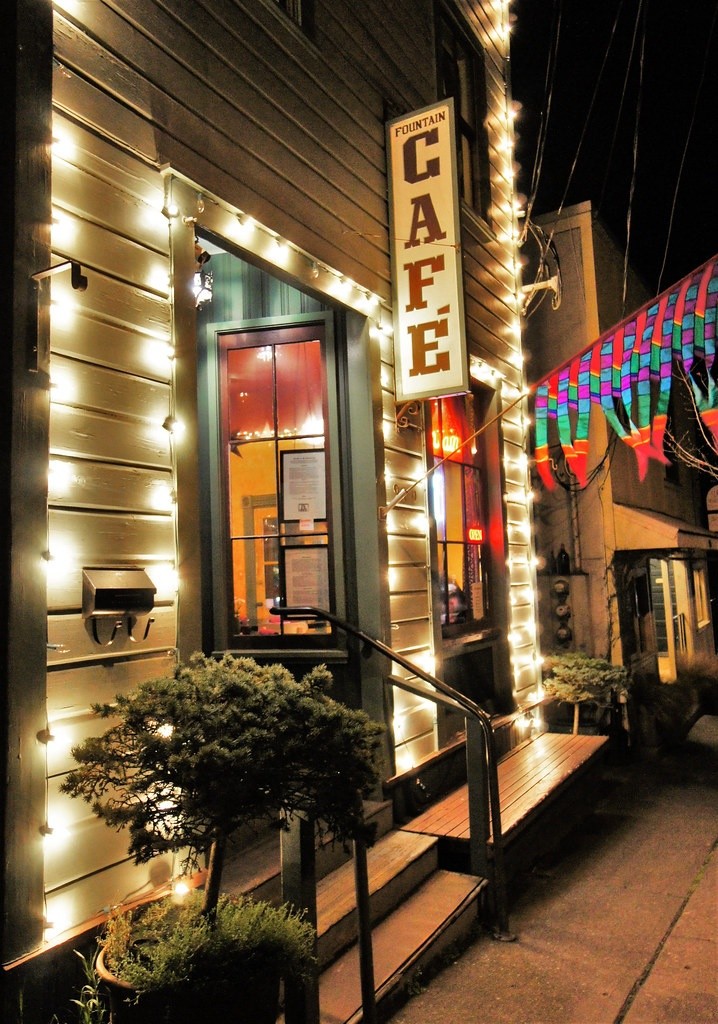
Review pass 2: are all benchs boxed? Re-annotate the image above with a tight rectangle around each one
[401,734,616,910]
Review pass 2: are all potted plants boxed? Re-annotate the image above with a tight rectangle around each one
[540,649,632,794]
[60,650,385,1023]
